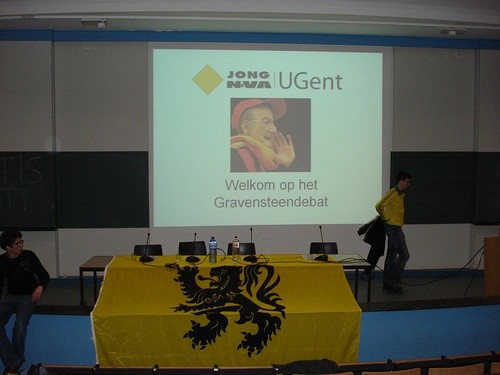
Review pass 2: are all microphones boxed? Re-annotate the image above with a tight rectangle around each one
[313,225,328,261]
[185,233,200,262]
[140,232,153,262]
[243,228,257,262]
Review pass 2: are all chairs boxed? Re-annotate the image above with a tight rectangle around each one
[178,240,207,255]
[37,352,500,375]
[309,242,338,254]
[133,244,163,256]
[227,243,255,255]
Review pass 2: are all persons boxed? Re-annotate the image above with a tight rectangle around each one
[0,229,50,375]
[375,173,413,295]
[230,98,304,172]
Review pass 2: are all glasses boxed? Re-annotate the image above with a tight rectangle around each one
[9,240,24,247]
[243,119,277,127]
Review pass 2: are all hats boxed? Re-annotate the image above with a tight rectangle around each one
[232,98,286,128]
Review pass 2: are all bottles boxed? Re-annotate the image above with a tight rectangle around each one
[209,236,217,263]
[232,236,240,261]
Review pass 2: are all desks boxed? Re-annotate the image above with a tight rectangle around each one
[79,254,372,307]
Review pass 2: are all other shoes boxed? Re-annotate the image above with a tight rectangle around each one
[3,358,24,375]
[383,281,402,295]
